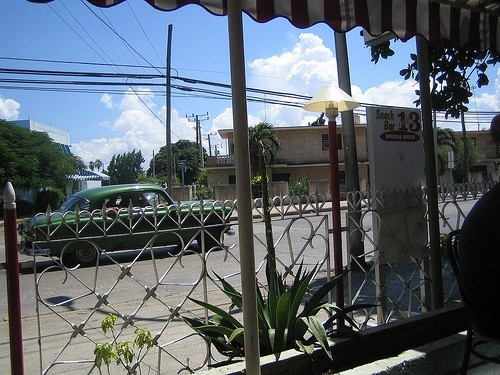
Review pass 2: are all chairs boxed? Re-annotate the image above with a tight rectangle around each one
[446,229,500,375]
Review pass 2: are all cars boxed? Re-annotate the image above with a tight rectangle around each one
[17,183,233,268]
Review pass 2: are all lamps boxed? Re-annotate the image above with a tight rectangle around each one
[302,86,361,122]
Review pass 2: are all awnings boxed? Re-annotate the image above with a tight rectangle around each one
[81,170,111,186]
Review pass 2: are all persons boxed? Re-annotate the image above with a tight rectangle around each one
[459,113,500,340]
[107,196,119,207]
